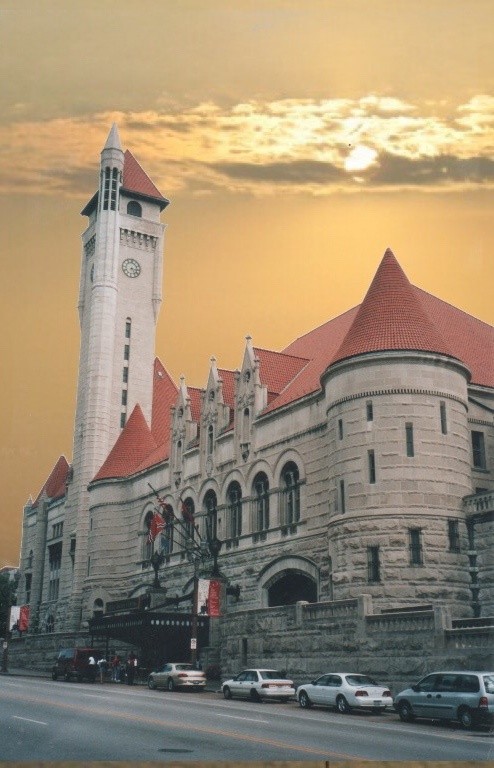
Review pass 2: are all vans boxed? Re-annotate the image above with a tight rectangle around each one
[52,648,100,683]
[394,671,494,728]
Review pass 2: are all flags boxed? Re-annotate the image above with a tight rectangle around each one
[209,579,221,619]
[8,605,21,632]
[144,512,167,546]
[179,501,197,526]
[18,607,30,633]
[155,495,175,529]
[197,578,210,617]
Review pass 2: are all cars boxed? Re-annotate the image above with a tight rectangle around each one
[148,662,207,692]
[220,668,295,703]
[296,673,393,714]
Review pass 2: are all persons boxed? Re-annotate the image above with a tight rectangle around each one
[84,650,142,687]
